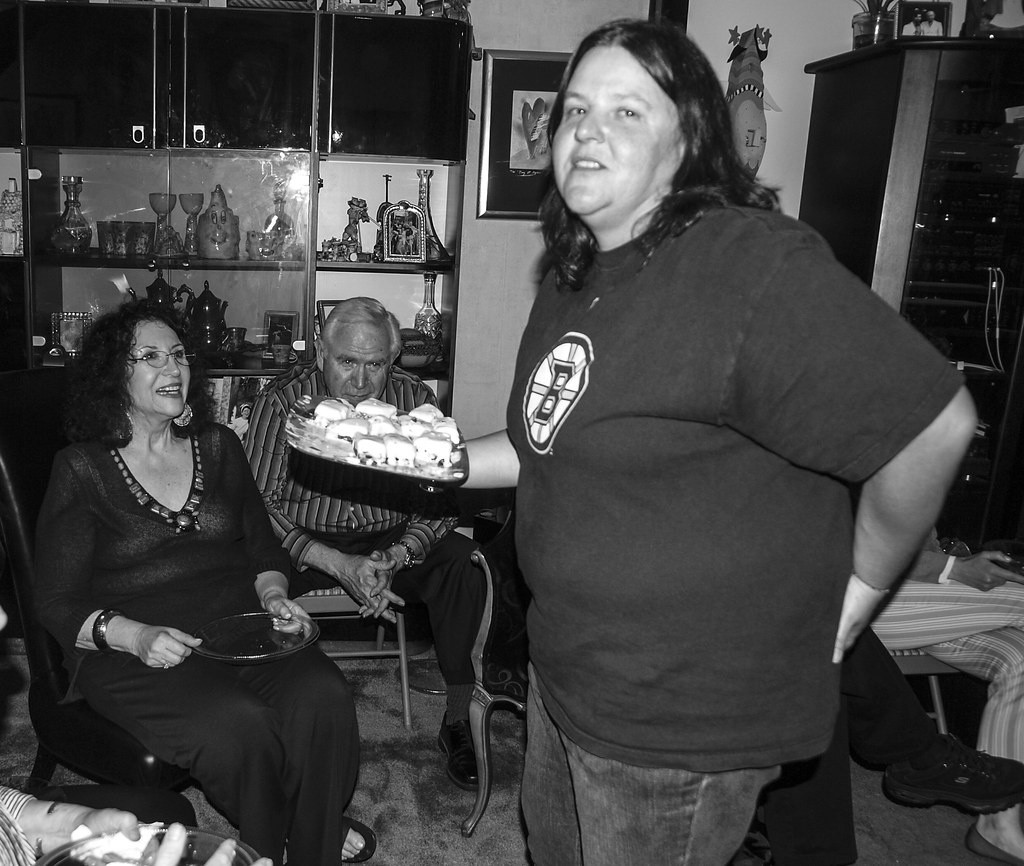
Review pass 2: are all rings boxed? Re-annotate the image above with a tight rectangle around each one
[163,662,170,669]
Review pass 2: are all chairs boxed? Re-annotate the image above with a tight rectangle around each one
[460,507,531,838]
[886,647,964,738]
[0,366,193,790]
[292,586,411,733]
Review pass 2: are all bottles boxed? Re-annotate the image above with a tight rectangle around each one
[263,182,295,259]
[51,175,92,254]
[414,270,444,362]
[0,178,23,255]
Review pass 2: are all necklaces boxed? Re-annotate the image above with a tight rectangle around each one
[585,234,655,313]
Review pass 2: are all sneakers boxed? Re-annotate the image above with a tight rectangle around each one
[883,731,1024,811]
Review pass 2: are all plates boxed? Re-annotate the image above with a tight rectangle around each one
[34,826,261,866]
[189,612,320,667]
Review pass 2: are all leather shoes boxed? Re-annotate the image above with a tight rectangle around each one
[437,707,479,791]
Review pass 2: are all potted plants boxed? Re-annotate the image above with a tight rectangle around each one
[851,0,898,50]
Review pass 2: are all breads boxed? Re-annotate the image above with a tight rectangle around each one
[313,395,462,468]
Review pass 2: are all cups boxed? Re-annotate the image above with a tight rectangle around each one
[272,345,297,364]
[223,327,247,356]
[249,231,279,262]
[97,220,156,256]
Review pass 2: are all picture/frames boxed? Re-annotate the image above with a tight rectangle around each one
[475,48,573,221]
[50,312,92,360]
[895,1,954,38]
[317,300,347,334]
[383,200,427,264]
[262,311,300,359]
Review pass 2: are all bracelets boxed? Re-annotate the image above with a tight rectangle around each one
[92,608,125,654]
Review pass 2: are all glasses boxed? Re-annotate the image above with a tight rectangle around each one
[125,339,197,368]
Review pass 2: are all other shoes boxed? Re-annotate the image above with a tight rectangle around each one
[966,826,1024,866]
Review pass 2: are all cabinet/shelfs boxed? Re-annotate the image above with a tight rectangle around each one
[796,36,1024,552]
[0,0,474,448]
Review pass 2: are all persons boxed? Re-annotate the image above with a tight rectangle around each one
[25,300,360,866]
[62,322,82,351]
[243,296,498,791]
[230,403,251,441]
[845,525,1024,866]
[902,11,943,36]
[455,17,977,866]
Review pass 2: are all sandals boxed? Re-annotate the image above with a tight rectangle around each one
[337,819,376,863]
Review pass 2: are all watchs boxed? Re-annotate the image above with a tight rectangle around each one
[392,540,416,568]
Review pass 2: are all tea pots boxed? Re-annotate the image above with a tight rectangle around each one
[188,280,228,352]
[125,269,194,325]
